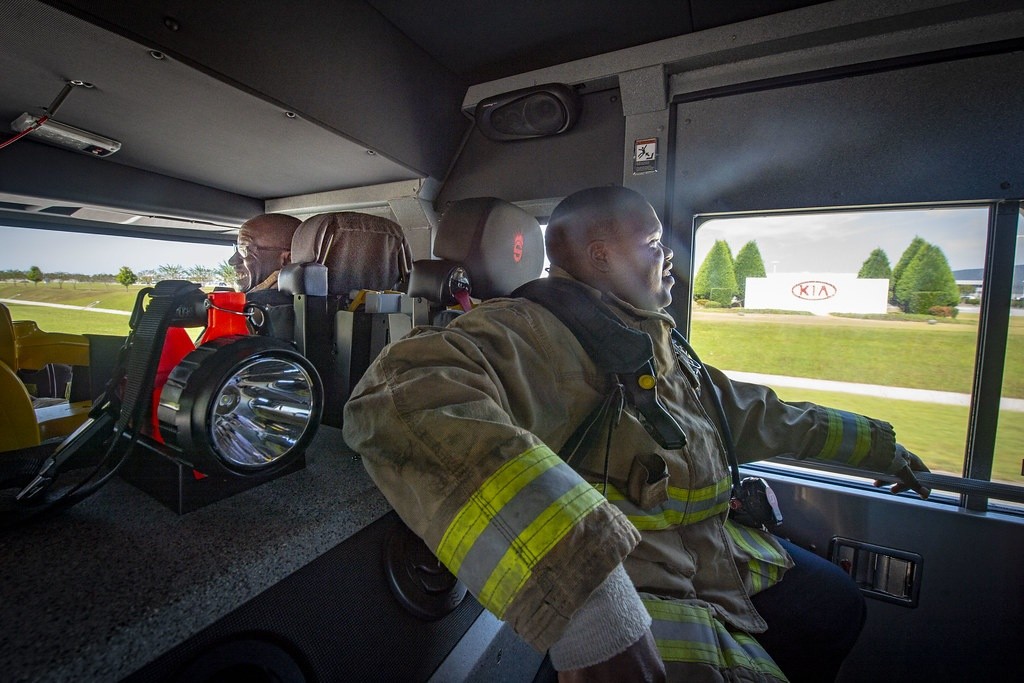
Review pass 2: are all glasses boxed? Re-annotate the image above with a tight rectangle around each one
[233,242,291,259]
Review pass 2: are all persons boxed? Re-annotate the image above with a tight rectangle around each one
[344,186,933,683]
[228,213,303,295]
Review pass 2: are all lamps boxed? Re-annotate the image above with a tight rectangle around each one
[158,334,325,480]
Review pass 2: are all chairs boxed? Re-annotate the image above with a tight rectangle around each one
[246,211,412,419]
[389,196,545,348]
